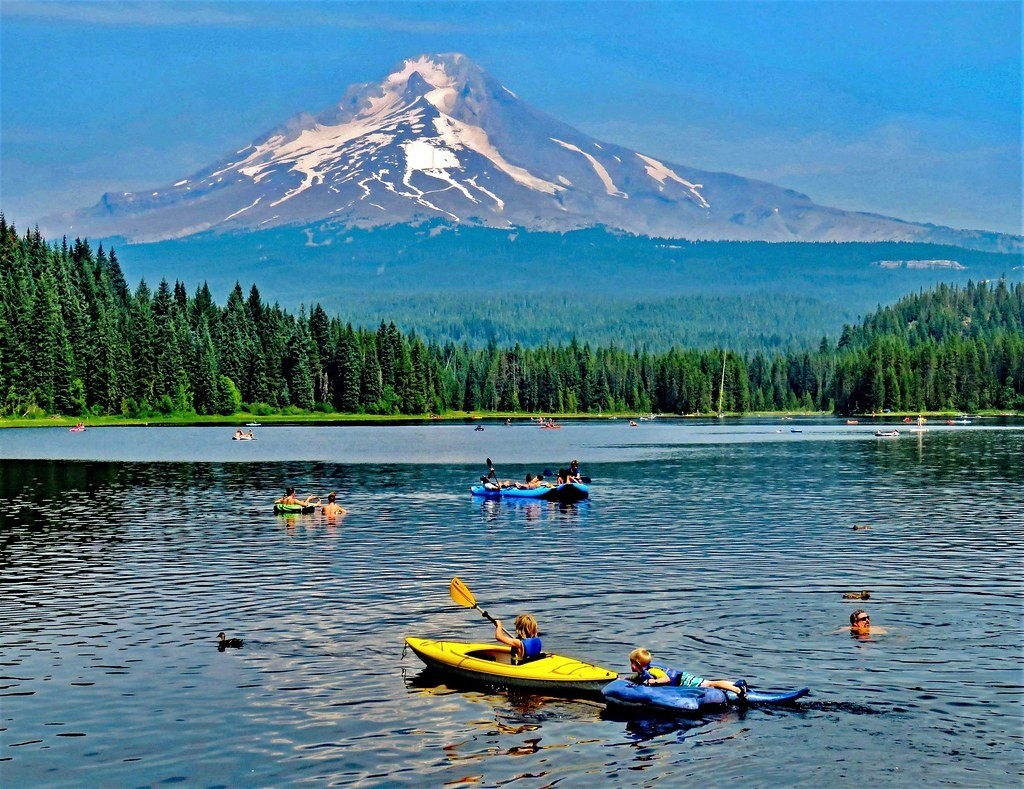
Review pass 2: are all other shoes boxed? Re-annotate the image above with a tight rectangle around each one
[736,686,747,704]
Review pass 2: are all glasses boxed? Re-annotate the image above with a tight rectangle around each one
[855,616,870,621]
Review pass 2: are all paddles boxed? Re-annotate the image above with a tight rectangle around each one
[486,457,503,488]
[449,575,520,645]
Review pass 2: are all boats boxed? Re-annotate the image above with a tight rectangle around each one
[874,431,901,436]
[401,637,619,692]
[469,481,591,499]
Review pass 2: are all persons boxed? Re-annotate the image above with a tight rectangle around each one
[493,614,544,661]
[848,609,873,629]
[628,646,748,702]
[505,416,554,429]
[235,429,253,439]
[916,414,924,428]
[74,421,85,430]
[273,485,347,514]
[479,460,581,491]
[628,412,656,426]
[874,427,902,437]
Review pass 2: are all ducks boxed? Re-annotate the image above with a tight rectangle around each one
[216,631,244,645]
